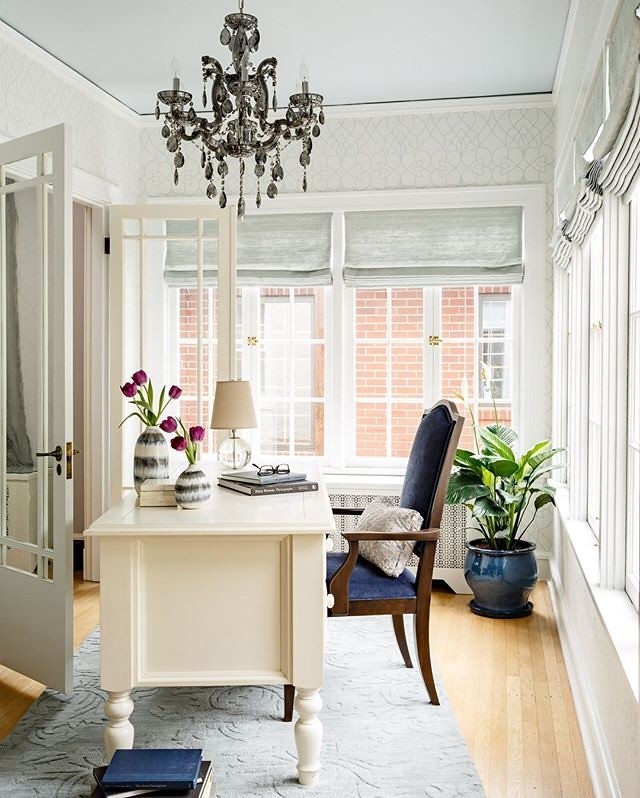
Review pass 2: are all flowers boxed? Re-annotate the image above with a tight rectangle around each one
[116,369,182,428]
[159,416,205,464]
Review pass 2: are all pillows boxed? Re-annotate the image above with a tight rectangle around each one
[353,498,424,578]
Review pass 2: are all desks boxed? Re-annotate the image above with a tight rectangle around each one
[83,466,335,784]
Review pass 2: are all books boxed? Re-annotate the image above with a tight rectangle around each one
[216,469,318,496]
[89,749,214,798]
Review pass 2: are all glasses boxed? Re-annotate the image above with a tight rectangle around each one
[252,464,290,476]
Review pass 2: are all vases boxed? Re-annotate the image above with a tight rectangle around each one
[174,464,212,510]
[134,426,170,496]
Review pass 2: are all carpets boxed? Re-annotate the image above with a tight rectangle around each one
[0,614,490,798]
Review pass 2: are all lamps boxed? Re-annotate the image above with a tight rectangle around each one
[154,0,325,218]
[210,380,258,469]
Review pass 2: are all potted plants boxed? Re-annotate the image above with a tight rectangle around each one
[444,368,569,619]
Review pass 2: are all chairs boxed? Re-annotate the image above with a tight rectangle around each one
[285,399,465,722]
